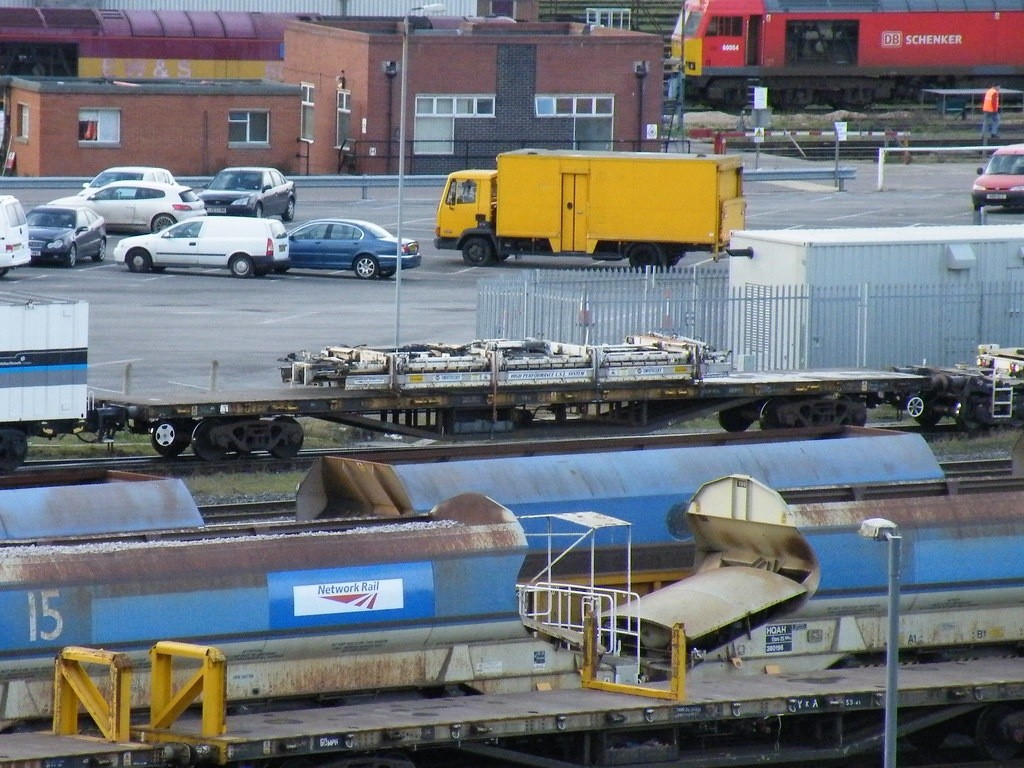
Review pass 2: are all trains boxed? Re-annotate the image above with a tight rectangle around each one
[0,6,329,118]
[670,0,1024,114]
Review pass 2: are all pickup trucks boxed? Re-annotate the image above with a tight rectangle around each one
[111,214,291,278]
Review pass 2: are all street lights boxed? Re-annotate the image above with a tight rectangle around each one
[862,512,909,768]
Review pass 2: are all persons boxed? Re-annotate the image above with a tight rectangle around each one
[980,81,1001,139]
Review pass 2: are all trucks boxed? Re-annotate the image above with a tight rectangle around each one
[432,146,748,273]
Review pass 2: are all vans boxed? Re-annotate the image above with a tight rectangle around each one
[970,143,1024,211]
[0,194,33,277]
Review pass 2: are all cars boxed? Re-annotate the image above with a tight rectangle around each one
[44,179,209,235]
[19,200,107,267]
[76,164,182,200]
[194,167,297,222]
[281,219,423,277]
